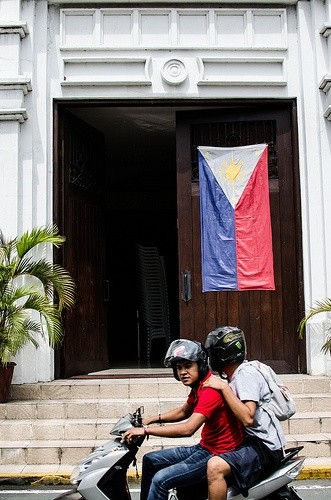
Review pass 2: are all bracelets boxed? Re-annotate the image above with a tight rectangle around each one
[159,414,161,424]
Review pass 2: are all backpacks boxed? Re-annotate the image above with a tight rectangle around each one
[236,360,297,422]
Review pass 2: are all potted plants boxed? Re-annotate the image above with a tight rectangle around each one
[0,223,74,403]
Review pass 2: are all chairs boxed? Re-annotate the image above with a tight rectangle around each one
[135,245,170,360]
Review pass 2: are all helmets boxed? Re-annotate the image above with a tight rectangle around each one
[205,326,246,372]
[163,339,208,381]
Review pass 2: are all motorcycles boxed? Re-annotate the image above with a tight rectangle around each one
[57,407,305,500]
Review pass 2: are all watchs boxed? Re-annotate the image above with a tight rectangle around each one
[143,425,149,441]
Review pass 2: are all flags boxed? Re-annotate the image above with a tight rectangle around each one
[198,145,276,292]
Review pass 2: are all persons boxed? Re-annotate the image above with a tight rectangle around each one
[122,339,248,500]
[205,326,286,500]
